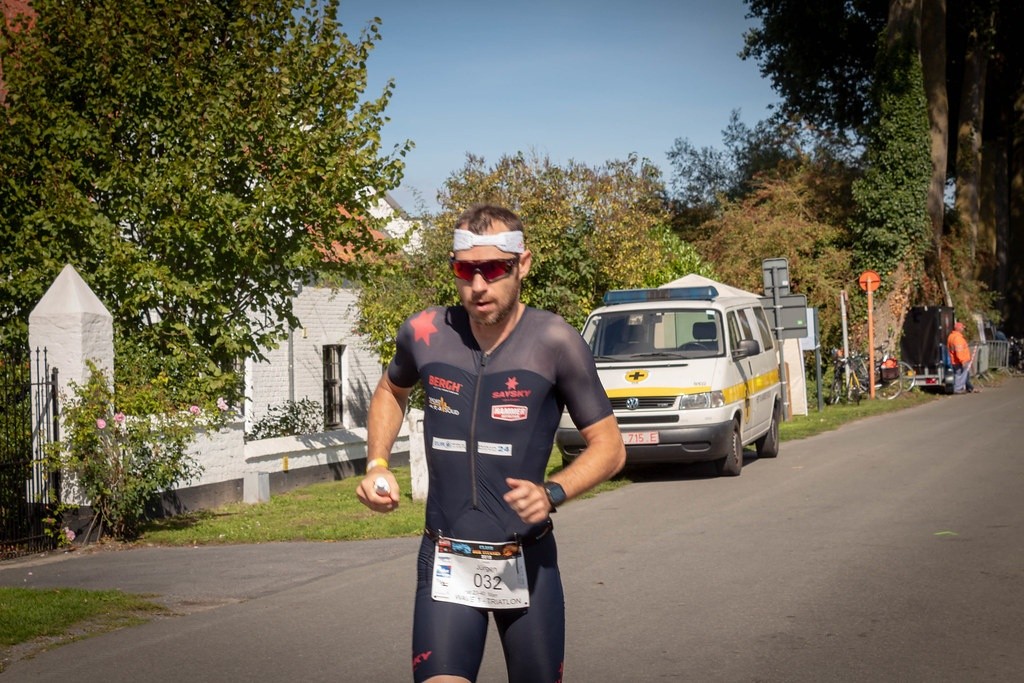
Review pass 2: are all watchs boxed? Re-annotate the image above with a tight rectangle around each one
[543,482,566,514]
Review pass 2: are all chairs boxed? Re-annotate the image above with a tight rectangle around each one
[685,322,718,350]
[611,325,654,355]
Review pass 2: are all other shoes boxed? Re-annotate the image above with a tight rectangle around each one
[970,389,981,393]
[954,390,967,394]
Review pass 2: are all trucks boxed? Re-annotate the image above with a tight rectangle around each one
[898,305,958,386]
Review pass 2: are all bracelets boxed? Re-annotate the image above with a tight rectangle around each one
[366,457,389,473]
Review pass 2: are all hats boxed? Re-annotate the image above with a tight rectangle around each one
[955,322,966,329]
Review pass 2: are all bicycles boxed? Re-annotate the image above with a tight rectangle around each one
[828,347,917,406]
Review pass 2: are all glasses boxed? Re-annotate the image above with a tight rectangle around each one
[449,255,519,282]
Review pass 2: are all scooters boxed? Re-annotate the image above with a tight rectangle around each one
[995,331,1024,374]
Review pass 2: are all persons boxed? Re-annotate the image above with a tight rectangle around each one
[356,206,626,683]
[947,323,971,395]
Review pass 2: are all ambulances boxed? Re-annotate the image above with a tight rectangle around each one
[557,285,785,476]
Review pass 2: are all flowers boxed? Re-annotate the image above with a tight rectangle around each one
[41,398,231,542]
